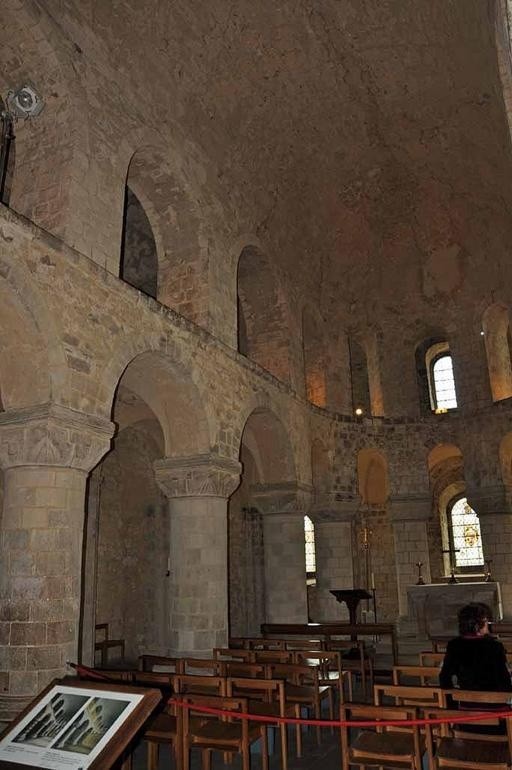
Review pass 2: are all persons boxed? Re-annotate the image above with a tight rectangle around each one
[438,603,512,736]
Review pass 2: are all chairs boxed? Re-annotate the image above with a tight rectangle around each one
[65,621,512,770]
